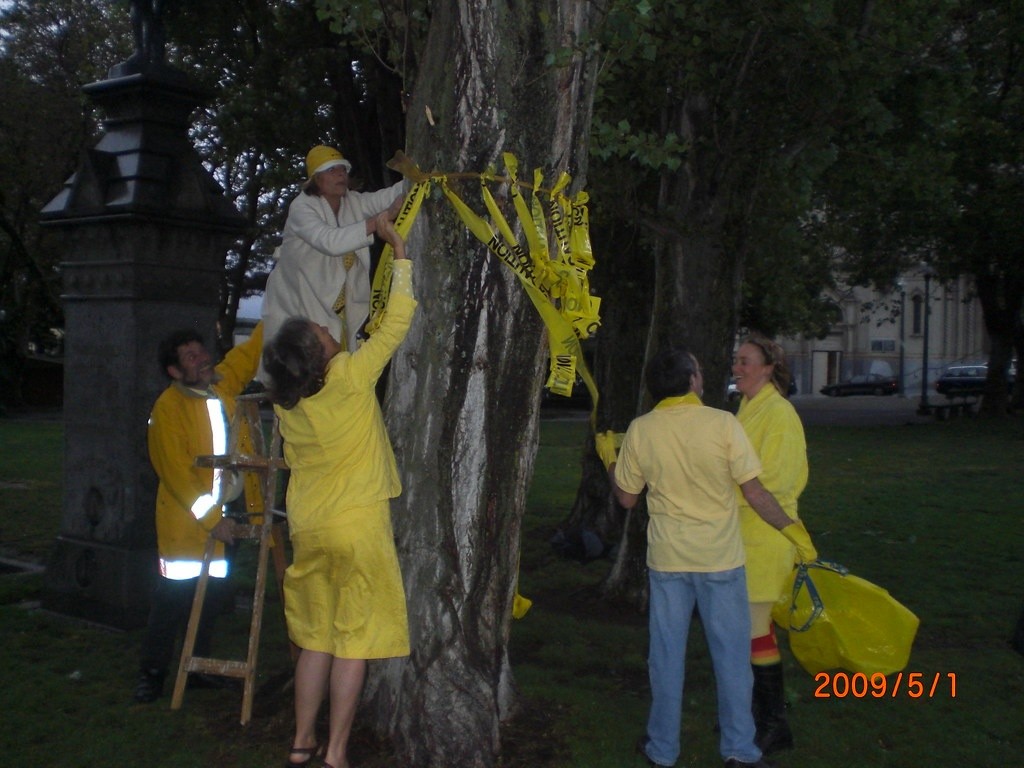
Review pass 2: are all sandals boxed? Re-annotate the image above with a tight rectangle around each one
[288,741,328,768]
[319,761,334,768]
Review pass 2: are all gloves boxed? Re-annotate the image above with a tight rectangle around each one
[595,430,618,471]
[779,519,818,566]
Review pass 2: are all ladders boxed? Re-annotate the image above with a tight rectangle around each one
[170,392,302,725]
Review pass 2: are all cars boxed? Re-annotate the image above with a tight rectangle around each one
[971,359,1018,374]
[820,373,898,397]
[726,371,797,402]
[242,378,266,409]
[936,365,1017,398]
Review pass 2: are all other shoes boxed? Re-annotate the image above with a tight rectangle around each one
[753,717,793,755]
[637,734,675,768]
[726,758,780,768]
[188,671,223,690]
[135,668,165,702]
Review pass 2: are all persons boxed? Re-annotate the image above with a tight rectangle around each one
[261,212,418,768]
[594,348,818,768]
[734,335,807,753]
[134,319,261,704]
[255,145,405,391]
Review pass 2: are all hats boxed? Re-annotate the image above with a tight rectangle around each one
[299,145,351,189]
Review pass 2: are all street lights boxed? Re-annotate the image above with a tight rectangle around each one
[898,276,908,397]
[918,257,936,415]
